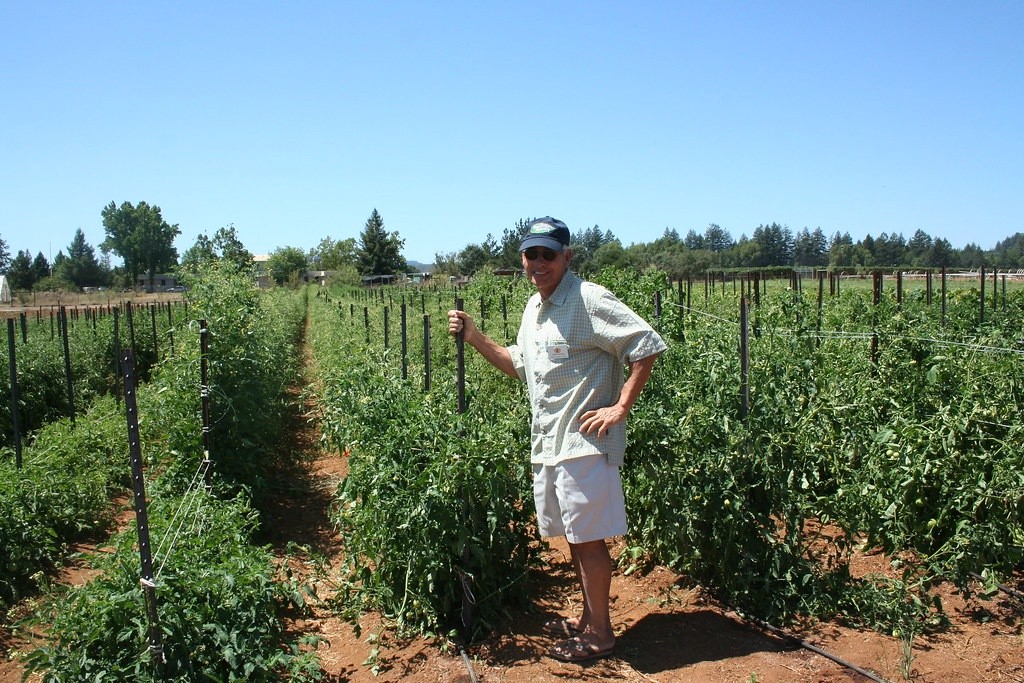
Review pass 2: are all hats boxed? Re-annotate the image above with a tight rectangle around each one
[518,216,570,252]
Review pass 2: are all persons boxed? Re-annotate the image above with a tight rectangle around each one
[446,216,668,663]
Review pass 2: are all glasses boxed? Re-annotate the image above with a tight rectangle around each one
[521,247,566,261]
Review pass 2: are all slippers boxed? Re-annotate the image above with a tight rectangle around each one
[548,636,614,660]
[538,618,583,639]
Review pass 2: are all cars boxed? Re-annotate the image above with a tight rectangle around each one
[166,285,185,293]
